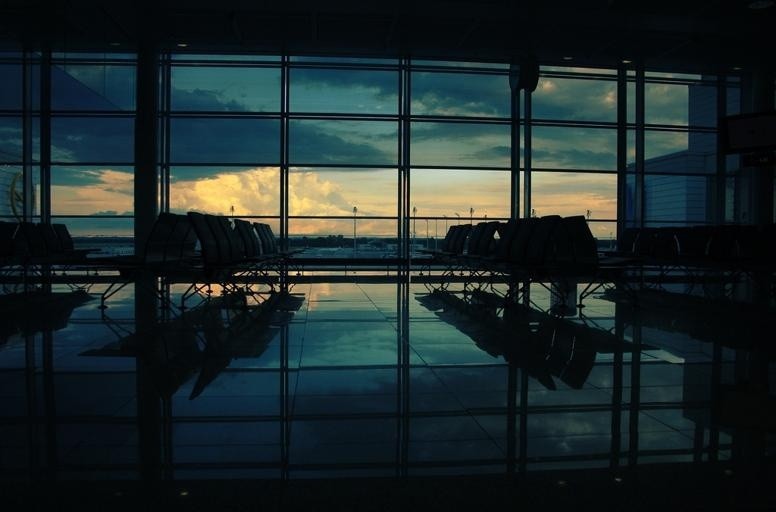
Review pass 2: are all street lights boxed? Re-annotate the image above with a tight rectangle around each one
[411,205,417,244]
[530,208,536,217]
[424,207,488,251]
[350,206,357,258]
[585,209,592,227]
[229,205,235,217]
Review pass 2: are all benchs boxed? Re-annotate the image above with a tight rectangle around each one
[94,212,305,309]
[0,223,101,295]
[0,290,94,348]
[86,292,308,357]
[595,293,769,355]
[625,225,776,297]
[414,289,657,366]
[422,215,638,287]
